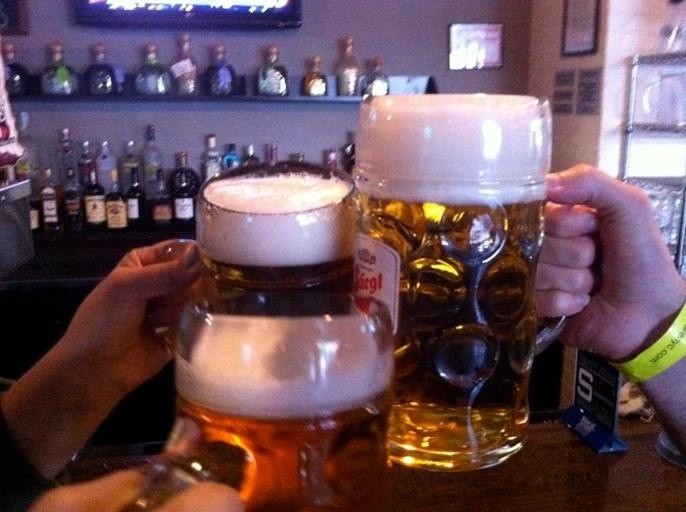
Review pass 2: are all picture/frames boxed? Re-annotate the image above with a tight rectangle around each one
[560,0,598,56]
[447,20,506,72]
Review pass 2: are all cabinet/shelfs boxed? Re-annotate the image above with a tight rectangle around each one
[0,73,436,238]
[620,53,686,295]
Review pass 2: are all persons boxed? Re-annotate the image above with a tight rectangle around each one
[0,239,203,511]
[534,164,686,456]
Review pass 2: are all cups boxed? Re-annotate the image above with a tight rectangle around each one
[350,89,590,474]
[133,158,395,511]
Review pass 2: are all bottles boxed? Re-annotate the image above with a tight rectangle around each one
[174,34,200,96]
[14,109,357,236]
[84,43,117,96]
[131,44,172,97]
[2,41,32,98]
[40,41,80,96]
[336,36,360,96]
[204,44,235,96]
[257,46,288,96]
[367,55,388,95]
[303,56,327,96]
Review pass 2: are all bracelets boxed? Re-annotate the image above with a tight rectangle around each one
[608,303,686,383]
